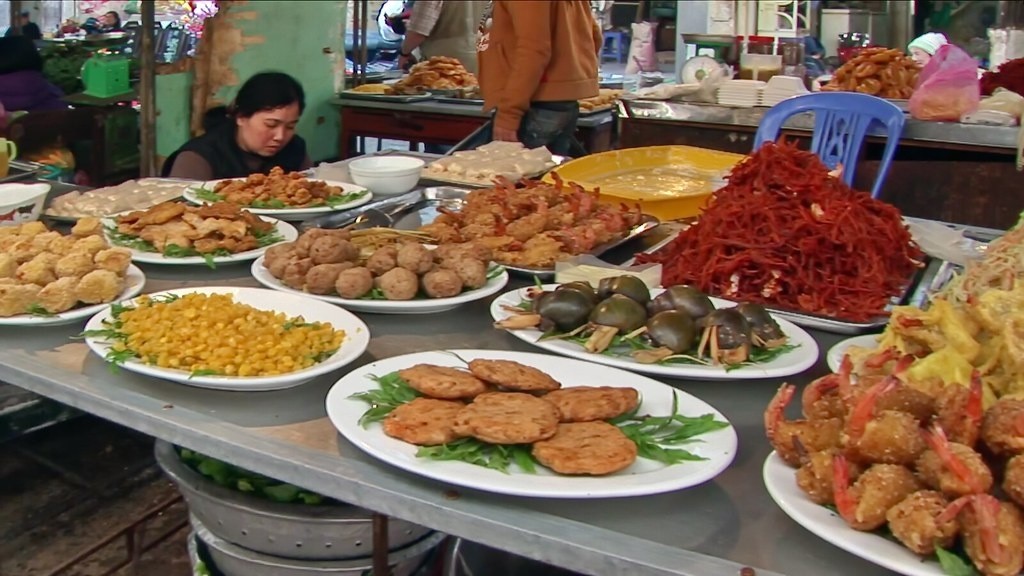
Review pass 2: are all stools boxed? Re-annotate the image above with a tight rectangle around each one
[598,31,630,65]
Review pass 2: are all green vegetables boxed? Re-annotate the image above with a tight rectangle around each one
[517,276,802,371]
[180,448,330,504]
[29,293,339,378]
[100,182,385,301]
[347,371,730,473]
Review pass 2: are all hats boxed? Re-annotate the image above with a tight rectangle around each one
[908,32,948,56]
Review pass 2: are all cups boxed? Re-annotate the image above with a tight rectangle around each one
[0,137,16,178]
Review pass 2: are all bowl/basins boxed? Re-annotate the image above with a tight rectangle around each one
[348,155,425,195]
[0,182,51,226]
[715,74,809,107]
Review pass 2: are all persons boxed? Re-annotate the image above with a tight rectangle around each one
[4,11,43,39]
[57,11,120,38]
[160,71,313,182]
[398,0,490,154]
[481,0,604,157]
[907,31,952,68]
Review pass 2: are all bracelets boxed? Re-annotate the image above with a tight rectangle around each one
[399,47,412,57]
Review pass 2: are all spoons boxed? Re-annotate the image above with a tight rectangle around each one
[357,202,409,227]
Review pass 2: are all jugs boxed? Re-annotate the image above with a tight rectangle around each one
[738,53,783,83]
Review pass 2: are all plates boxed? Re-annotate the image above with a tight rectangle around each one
[761,450,949,576]
[100,209,298,266]
[251,243,509,315]
[0,263,146,326]
[826,333,883,385]
[182,177,373,221]
[83,286,371,391]
[489,284,819,380]
[325,348,738,499]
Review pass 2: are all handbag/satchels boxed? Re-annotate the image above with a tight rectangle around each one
[908,43,980,120]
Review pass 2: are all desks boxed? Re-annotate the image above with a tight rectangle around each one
[0,152,1002,575]
[62,83,144,185]
[49,32,129,55]
[326,98,615,163]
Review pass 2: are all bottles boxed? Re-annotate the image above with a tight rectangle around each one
[720,35,744,64]
[748,36,774,55]
[777,37,804,65]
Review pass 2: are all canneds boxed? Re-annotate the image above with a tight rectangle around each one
[747,36,806,66]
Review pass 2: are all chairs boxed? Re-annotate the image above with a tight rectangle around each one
[751,91,906,198]
[118,21,202,79]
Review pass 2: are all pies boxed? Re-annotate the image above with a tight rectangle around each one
[381,358,641,473]
[385,57,479,95]
